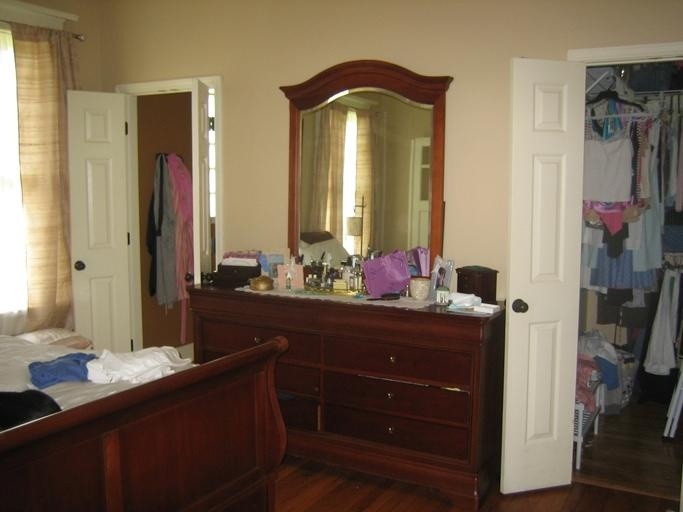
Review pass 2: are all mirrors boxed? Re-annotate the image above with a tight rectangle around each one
[279,60,454,278]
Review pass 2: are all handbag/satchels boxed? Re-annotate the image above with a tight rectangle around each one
[406,246,431,277]
[363,249,411,300]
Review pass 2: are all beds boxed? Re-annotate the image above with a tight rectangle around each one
[0,335,290,512]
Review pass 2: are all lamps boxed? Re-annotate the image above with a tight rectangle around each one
[347,196,366,256]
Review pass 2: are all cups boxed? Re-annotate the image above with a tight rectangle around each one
[410,278,430,301]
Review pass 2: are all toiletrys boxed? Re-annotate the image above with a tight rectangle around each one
[305,261,365,293]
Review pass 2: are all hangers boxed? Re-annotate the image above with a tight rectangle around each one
[586,76,652,121]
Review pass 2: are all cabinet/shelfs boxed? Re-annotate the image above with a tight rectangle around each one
[186,283,504,512]
[574,382,601,470]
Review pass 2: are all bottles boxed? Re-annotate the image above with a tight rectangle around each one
[284,265,293,293]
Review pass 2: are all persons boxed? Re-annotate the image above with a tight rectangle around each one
[436,268,450,291]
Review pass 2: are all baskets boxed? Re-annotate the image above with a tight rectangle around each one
[615,349,640,409]
